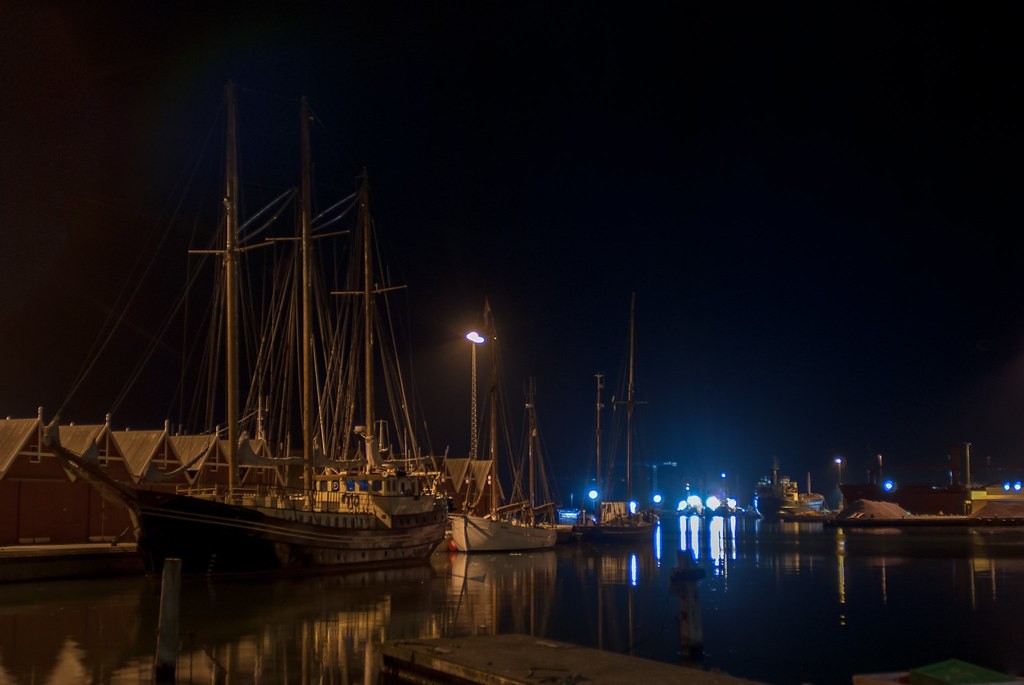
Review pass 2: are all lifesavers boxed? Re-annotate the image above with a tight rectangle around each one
[448,540,457,553]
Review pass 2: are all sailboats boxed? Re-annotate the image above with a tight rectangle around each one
[38,85,557,574]
[567,291,660,546]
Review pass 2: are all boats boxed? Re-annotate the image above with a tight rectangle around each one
[755,464,825,519]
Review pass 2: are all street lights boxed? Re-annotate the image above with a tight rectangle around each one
[466,331,485,458]
[835,458,841,484]
